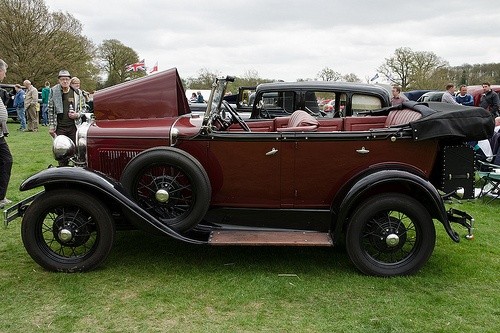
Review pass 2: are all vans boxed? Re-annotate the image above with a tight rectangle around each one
[1,84,30,121]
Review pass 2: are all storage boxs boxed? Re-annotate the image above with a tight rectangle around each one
[433,143,475,199]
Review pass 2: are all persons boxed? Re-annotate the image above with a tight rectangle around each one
[0,59,13,206]
[390,87,407,107]
[46,70,81,146]
[188,90,203,104]
[478,83,499,115]
[456,86,474,106]
[14,69,98,133]
[441,85,464,106]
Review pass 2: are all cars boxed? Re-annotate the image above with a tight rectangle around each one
[3,65,470,274]
[401,80,498,105]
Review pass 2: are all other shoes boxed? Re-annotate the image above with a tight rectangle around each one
[0,196,12,204]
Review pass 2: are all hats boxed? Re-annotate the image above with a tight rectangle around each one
[58,69,71,78]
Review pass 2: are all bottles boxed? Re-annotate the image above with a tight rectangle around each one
[68,104,74,112]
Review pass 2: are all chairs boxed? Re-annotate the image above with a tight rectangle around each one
[478,159,500,206]
[270,110,320,135]
[369,108,422,132]
